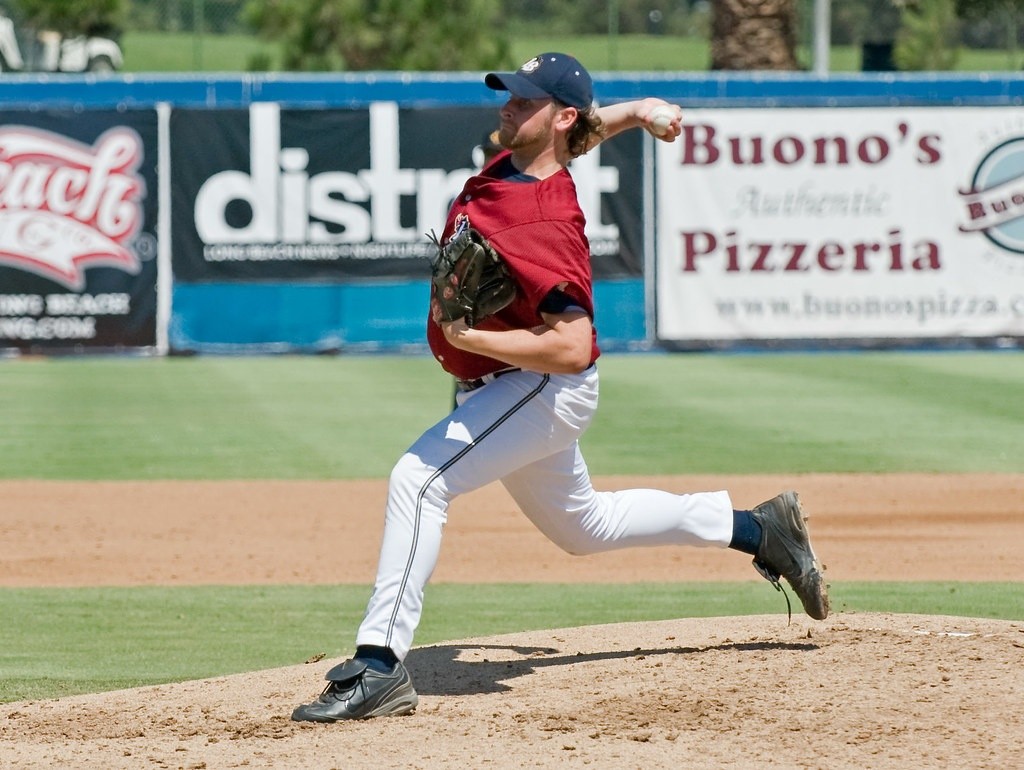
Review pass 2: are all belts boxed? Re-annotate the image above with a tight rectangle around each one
[457,368,521,392]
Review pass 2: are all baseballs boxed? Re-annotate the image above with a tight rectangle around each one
[647,105,678,137]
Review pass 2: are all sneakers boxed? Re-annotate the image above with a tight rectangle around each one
[750,490,831,627]
[291,659,418,723]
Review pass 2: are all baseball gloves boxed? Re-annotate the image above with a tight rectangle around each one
[427,226,520,329]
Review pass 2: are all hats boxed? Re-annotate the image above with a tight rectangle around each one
[484,52,593,109]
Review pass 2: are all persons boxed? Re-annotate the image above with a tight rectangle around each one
[289,55,830,725]
